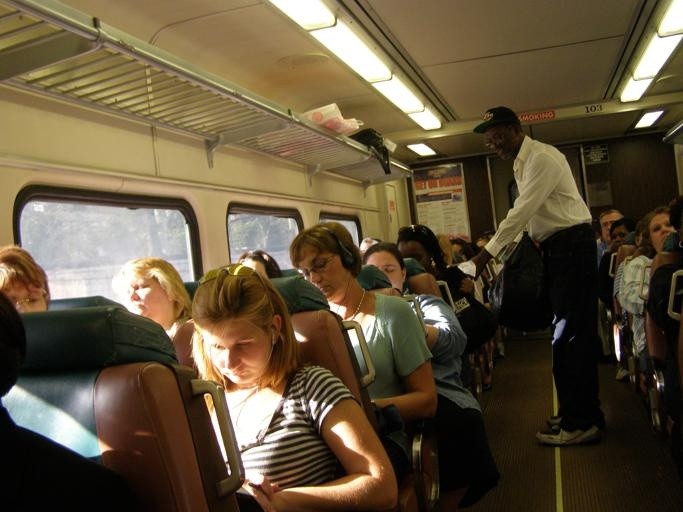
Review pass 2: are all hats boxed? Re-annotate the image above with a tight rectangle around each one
[473,106,518,132]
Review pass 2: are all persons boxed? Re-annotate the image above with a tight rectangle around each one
[0,224,507,512]
[469,107,606,446]
[595,196,683,485]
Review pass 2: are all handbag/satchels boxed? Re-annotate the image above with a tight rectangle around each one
[348,128,383,147]
[493,232,548,332]
[258,103,364,157]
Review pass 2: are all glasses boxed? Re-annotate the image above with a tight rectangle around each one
[301,255,334,279]
[9,290,47,309]
[198,265,276,314]
[485,132,509,149]
[397,225,429,239]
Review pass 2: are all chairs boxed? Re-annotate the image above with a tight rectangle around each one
[19,309,248,511]
[266,275,421,510]
[604,243,683,452]
[276,270,299,277]
[280,258,499,401]
[183,281,199,303]
[47,294,130,312]
[357,265,444,511]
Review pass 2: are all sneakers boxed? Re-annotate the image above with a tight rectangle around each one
[616,368,629,381]
[535,413,606,445]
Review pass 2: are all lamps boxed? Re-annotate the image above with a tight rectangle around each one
[268,0,442,131]
[620,0,683,102]
